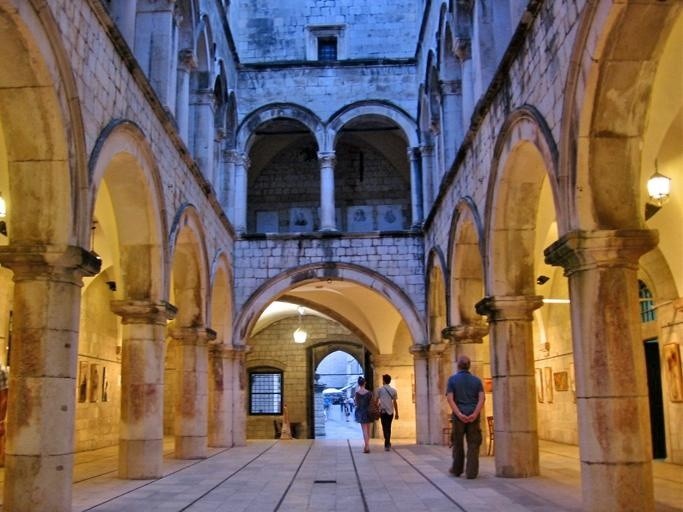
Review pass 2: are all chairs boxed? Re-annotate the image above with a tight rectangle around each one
[442,414,452,446]
[486,415,494,455]
[273,420,281,438]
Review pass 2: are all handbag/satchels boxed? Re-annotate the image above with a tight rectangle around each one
[367,399,379,421]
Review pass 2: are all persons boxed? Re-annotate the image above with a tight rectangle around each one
[373,372,399,453]
[444,355,486,479]
[353,375,376,454]
[322,392,354,422]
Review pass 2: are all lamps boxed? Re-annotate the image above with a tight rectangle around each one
[0,190,10,220]
[292,305,308,343]
[646,155,671,201]
[88,217,101,260]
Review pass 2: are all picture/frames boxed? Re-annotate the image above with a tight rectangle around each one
[660,341,683,406]
[77,359,110,404]
[534,361,576,406]
[254,204,406,235]
[482,377,494,394]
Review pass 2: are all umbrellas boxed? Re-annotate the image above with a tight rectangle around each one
[322,387,342,393]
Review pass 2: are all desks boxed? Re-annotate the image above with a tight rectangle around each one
[279,422,300,438]
